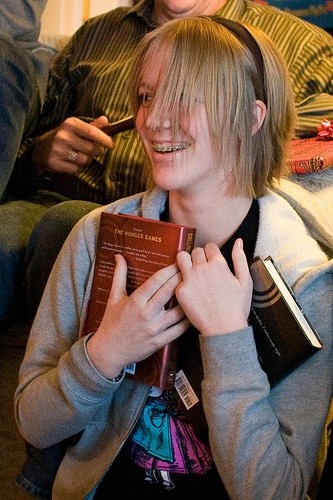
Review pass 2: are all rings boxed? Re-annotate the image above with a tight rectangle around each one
[67,149,79,163]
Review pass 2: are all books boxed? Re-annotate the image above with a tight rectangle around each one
[171,255,325,440]
[83,211,196,391]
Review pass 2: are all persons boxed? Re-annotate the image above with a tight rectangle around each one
[11,0,333,318]
[13,10,333,500]
